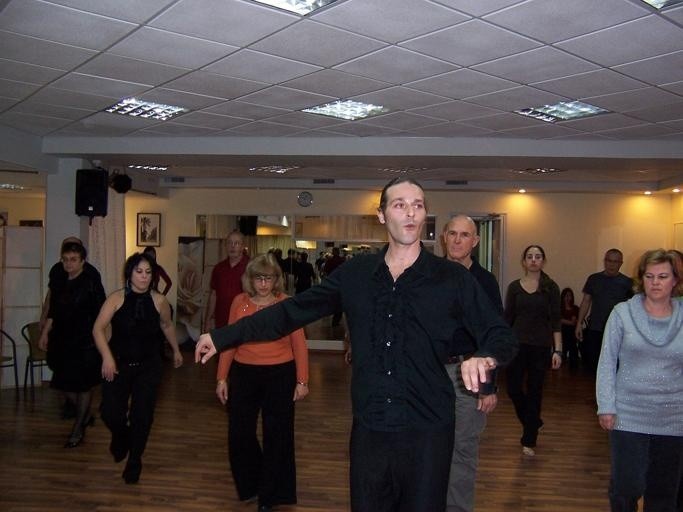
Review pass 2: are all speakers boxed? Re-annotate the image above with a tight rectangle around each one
[75,168,107,225]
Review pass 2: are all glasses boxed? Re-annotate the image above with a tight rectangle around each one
[226,240,243,247]
[253,277,273,282]
[60,257,80,263]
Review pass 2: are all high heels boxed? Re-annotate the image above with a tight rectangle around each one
[64,432,86,447]
[88,415,96,426]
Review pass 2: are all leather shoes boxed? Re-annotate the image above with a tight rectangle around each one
[114,442,130,463]
[125,465,142,484]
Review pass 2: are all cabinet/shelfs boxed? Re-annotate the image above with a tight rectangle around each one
[0,222,45,389]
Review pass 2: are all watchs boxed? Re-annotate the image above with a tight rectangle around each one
[553,350,563,355]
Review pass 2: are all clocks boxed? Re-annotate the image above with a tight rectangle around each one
[297,191,313,207]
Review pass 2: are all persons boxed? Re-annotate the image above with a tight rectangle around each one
[300,252,317,283]
[575,249,636,379]
[323,247,346,326]
[275,248,284,266]
[39,244,106,447]
[94,252,184,485]
[50,235,101,284]
[204,232,252,335]
[314,252,324,272]
[217,252,309,512]
[133,248,173,296]
[196,177,516,512]
[438,214,506,510]
[598,249,683,512]
[507,245,564,456]
[279,248,317,298]
[562,289,580,360]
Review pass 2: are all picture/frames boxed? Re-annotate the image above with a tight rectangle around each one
[136,212,161,248]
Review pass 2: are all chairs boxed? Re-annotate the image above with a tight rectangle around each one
[0,330,20,399]
[21,320,56,406]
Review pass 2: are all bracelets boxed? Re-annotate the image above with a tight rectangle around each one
[296,382,309,387]
[216,380,228,385]
